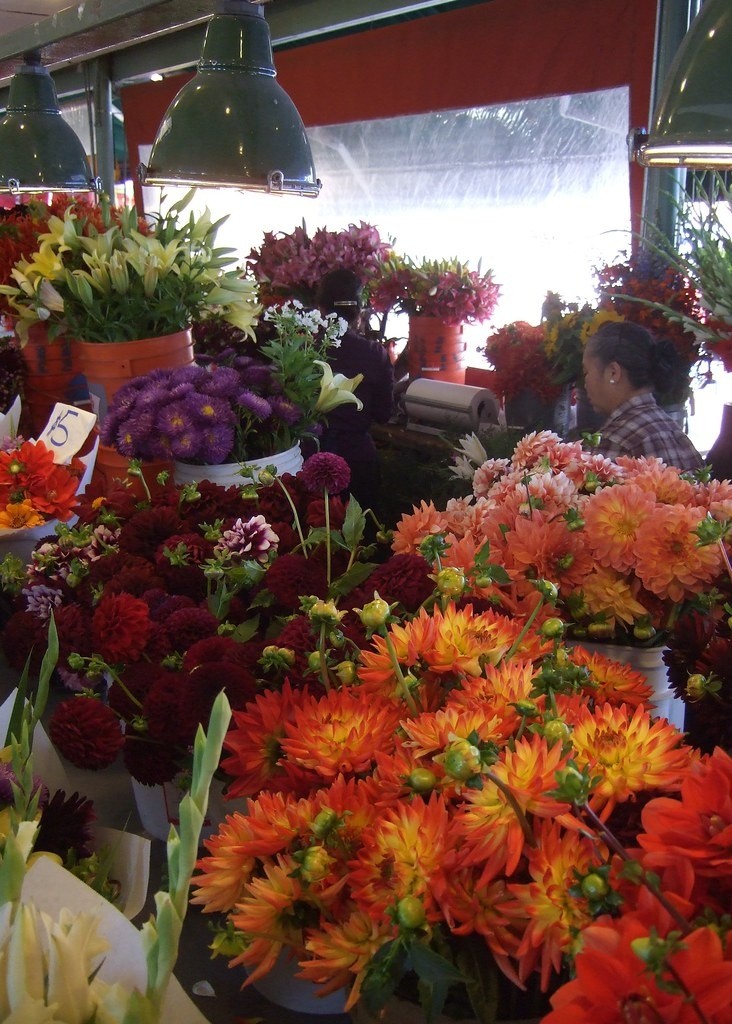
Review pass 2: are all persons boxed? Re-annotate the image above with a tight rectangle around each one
[300,283,399,532]
[581,320,710,485]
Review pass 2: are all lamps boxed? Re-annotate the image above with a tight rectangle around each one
[0,49,101,196]
[135,0,323,198]
[637,0,732,170]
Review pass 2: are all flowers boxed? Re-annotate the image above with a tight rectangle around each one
[0,167,732,1024]
